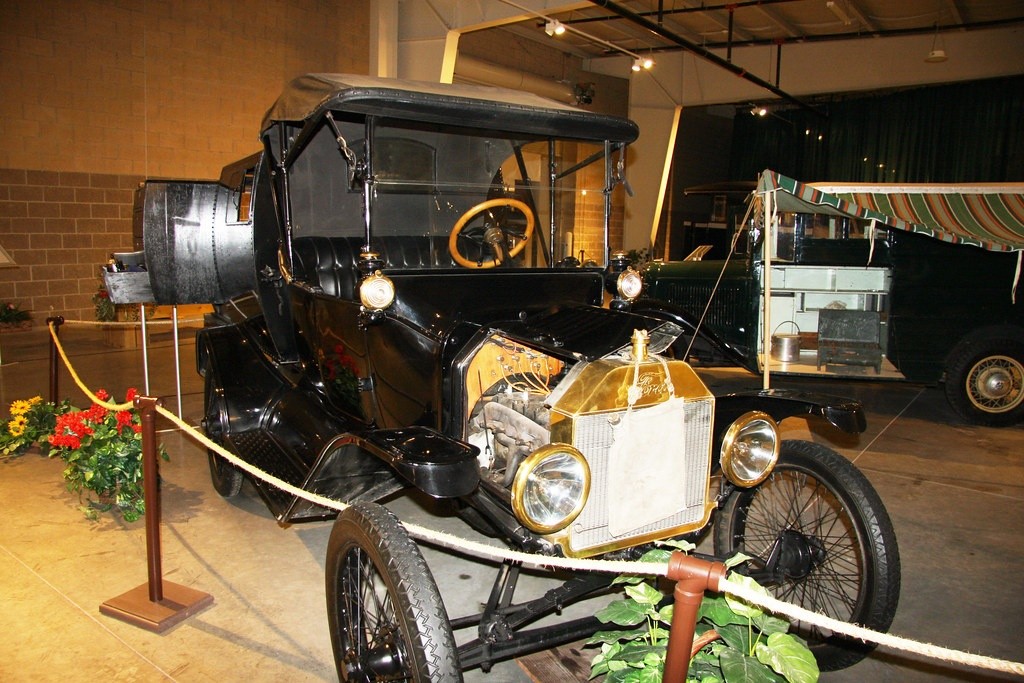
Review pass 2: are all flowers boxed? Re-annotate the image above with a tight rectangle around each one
[49,387,170,523]
[92,280,161,324]
[319,344,367,418]
[0,393,72,462]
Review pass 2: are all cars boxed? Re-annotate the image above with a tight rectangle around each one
[101,74,901,683]
[605,169,1024,427]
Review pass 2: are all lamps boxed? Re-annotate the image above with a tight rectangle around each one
[750,107,767,117]
[632,57,654,73]
[543,18,566,37]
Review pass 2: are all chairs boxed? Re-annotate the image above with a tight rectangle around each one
[816,308,883,374]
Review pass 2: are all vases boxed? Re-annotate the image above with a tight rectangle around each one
[97,480,142,505]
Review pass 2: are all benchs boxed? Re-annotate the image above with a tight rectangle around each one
[290,231,518,300]
